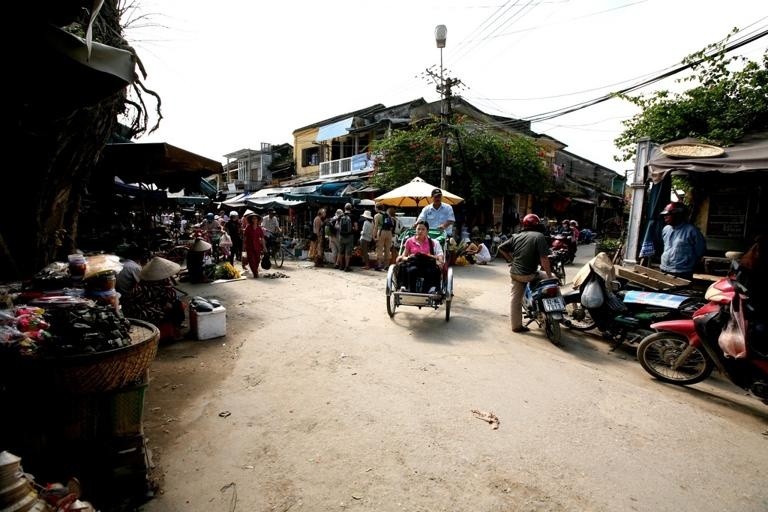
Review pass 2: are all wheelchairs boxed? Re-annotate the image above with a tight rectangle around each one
[386,228,454,321]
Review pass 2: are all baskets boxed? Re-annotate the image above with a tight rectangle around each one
[46,317,161,393]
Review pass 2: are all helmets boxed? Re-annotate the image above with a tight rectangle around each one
[660,201,688,216]
[562,220,568,225]
[523,213,541,224]
[230,210,238,217]
[206,213,214,220]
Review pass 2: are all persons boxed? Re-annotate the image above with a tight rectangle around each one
[660,204,708,282]
[118,257,188,346]
[497,214,557,333]
[114,245,148,293]
[161,189,581,296]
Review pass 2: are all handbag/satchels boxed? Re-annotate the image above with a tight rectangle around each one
[261,253,271,269]
[307,233,317,242]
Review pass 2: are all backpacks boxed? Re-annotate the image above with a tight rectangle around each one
[340,214,353,237]
[325,218,337,236]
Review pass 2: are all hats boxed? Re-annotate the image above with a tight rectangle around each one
[360,211,373,220]
[191,240,211,252]
[138,257,180,281]
[243,208,263,224]
[345,202,351,209]
[432,189,442,196]
[570,220,579,227]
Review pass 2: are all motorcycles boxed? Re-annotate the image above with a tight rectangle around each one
[561,252,704,366]
[637,257,768,404]
[522,278,565,343]
[491,232,508,258]
[549,233,573,265]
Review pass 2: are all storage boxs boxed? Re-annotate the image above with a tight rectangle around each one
[189,302,226,341]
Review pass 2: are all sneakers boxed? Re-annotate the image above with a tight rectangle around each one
[400,286,408,291]
[512,327,529,332]
[335,264,352,273]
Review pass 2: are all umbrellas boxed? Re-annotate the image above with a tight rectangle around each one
[374,175,464,216]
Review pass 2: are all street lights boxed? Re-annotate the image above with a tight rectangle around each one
[435,24,448,191]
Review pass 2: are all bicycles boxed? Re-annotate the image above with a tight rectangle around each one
[266,233,284,268]
